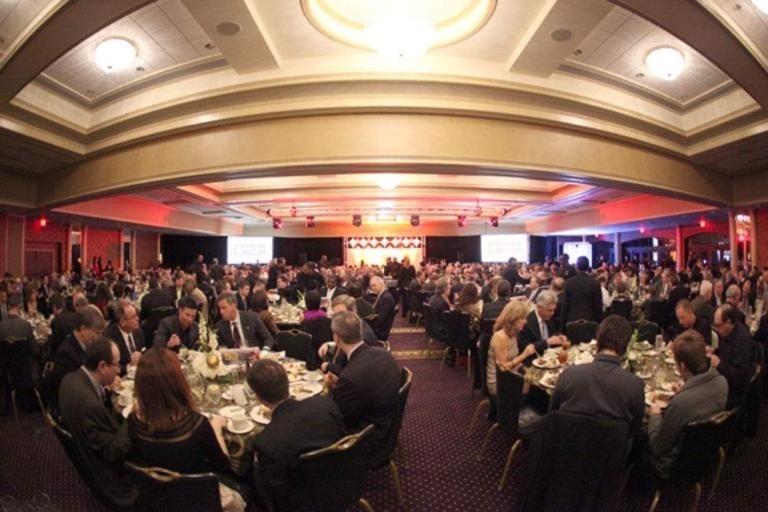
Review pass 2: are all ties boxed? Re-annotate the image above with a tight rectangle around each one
[128,335,135,353]
[233,322,242,348]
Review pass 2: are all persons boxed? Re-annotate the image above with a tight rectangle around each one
[323,310,400,461]
[383,253,768,494]
[110,346,245,512]
[1,254,383,410]
[59,336,139,512]
[245,359,347,508]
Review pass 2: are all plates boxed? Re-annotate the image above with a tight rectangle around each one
[533,346,680,408]
[118,346,323,435]
